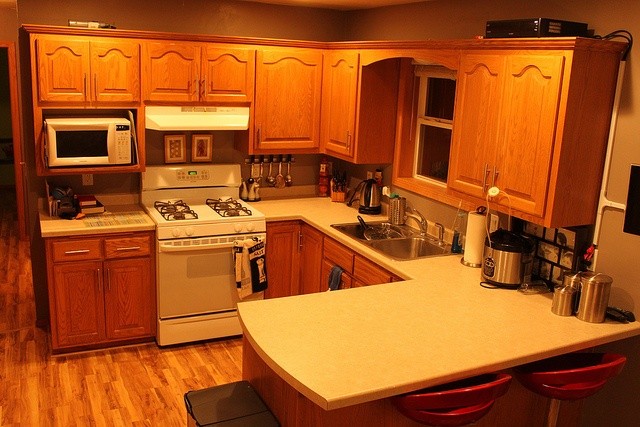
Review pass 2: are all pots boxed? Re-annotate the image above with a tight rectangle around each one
[357,215,404,241]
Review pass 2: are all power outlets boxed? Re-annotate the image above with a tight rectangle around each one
[82,174,94,186]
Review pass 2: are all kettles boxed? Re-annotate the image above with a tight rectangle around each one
[359,179,381,215]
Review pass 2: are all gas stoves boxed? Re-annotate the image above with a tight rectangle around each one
[139,197,266,227]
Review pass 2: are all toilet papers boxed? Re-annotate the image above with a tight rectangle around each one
[463,211,489,264]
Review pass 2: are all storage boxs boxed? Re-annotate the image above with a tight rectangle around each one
[173,379,283,426]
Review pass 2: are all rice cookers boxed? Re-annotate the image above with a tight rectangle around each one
[481,228,536,290]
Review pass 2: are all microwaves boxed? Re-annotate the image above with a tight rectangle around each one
[44,118,131,167]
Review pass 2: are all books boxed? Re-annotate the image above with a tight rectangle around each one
[80,199,105,214]
[77,194,96,206]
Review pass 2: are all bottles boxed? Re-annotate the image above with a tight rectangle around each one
[248,177,254,191]
[248,182,257,201]
[241,181,248,200]
[255,184,260,199]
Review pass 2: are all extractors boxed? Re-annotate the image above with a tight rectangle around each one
[144,107,249,131]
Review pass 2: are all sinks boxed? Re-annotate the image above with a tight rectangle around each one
[329,220,421,241]
[363,237,464,261]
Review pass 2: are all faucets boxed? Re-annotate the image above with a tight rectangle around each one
[403,209,428,237]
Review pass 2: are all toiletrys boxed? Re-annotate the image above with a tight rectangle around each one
[450,213,466,253]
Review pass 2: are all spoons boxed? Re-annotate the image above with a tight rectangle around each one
[265,154,275,184]
[285,154,292,184]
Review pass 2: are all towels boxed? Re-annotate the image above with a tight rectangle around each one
[244,238,268,293]
[328,264,344,291]
[232,237,252,300]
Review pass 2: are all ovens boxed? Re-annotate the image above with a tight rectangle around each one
[155,220,267,346]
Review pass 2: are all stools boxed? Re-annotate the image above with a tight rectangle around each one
[512,350,627,427]
[391,373,512,427]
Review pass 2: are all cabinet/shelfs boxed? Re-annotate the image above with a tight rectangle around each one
[322,40,401,165]
[33,105,146,177]
[352,255,393,288]
[447,36,629,228]
[143,30,252,105]
[299,224,323,295]
[264,220,298,299]
[20,24,141,105]
[324,235,356,292]
[45,230,157,355]
[253,38,322,150]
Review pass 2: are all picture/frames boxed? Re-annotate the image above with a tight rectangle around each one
[192,134,213,163]
[164,134,186,164]
[0,138,13,164]
